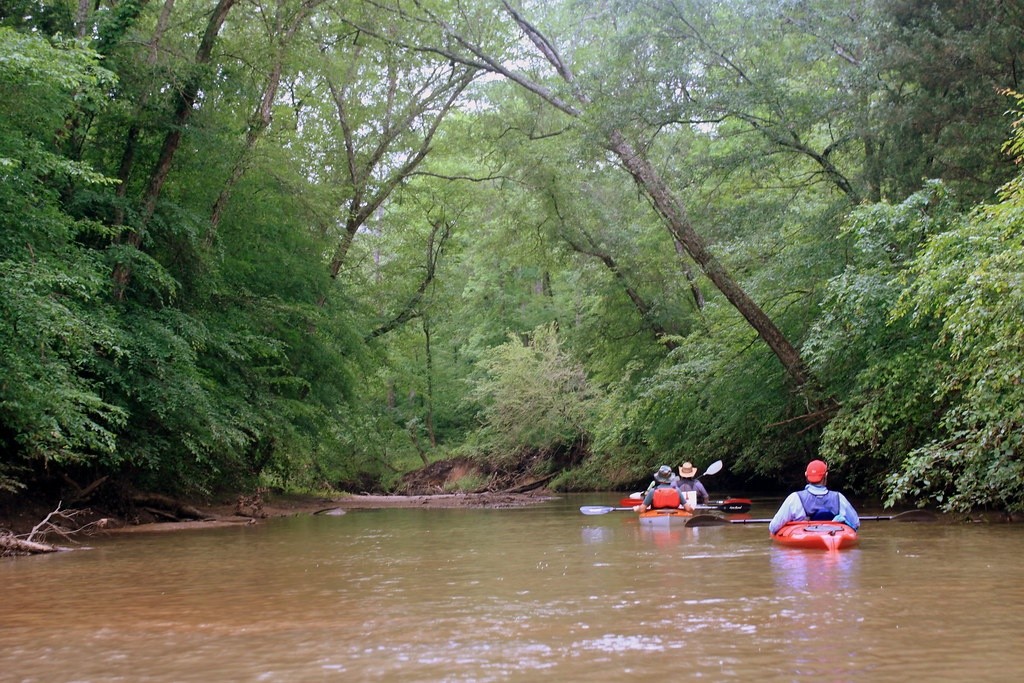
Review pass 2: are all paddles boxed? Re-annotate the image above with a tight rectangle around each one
[629,460,723,499]
[685,509,939,530]
[579,502,752,516]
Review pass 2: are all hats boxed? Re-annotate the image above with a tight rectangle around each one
[654,465,675,483]
[806,460,827,483]
[678,462,697,477]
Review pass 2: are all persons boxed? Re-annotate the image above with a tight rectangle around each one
[647,480,655,492]
[632,466,693,513]
[676,462,709,505]
[769,460,860,534]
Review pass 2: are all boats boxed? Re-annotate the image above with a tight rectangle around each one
[620,492,644,508]
[771,520,859,551]
[639,509,693,528]
